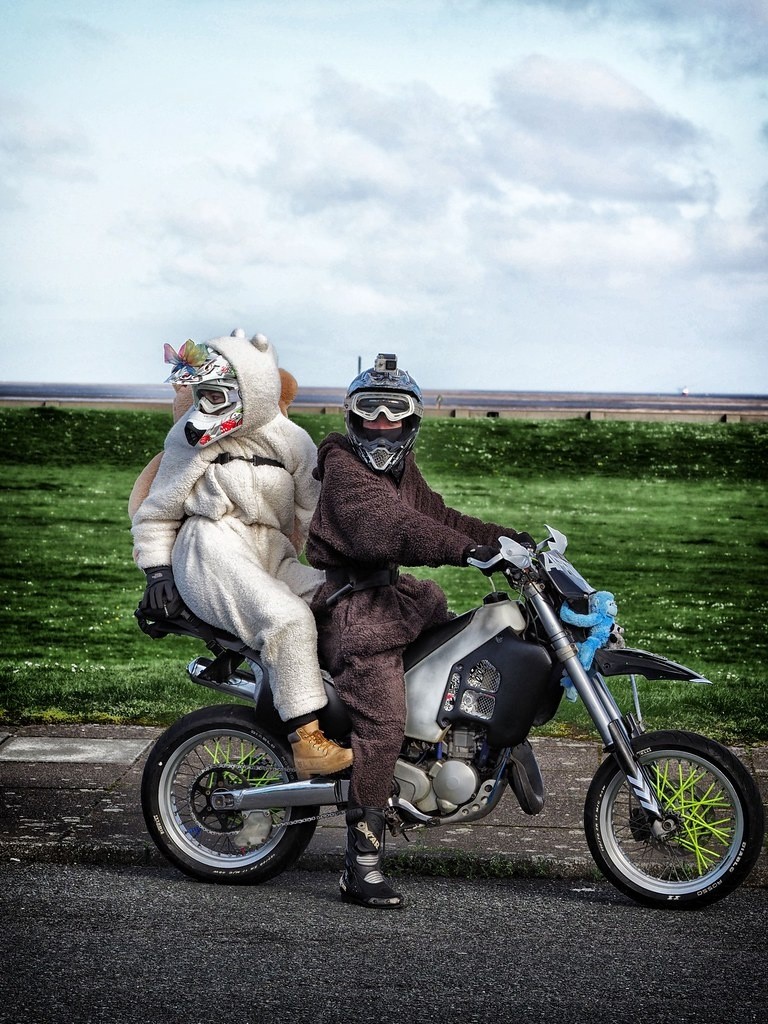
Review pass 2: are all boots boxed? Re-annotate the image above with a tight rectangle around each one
[338,805,405,909]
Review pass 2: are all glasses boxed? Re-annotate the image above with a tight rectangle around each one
[343,392,425,423]
[192,382,243,414]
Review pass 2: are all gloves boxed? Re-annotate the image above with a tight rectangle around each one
[512,532,537,554]
[465,544,515,576]
[140,566,175,610]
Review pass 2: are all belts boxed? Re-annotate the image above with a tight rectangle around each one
[326,561,398,596]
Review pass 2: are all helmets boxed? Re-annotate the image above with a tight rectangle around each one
[164,346,244,449]
[344,353,424,475]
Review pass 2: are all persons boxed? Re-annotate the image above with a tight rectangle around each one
[307,355,538,908]
[129,329,354,781]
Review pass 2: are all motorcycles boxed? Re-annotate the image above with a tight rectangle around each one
[132,524,767,913]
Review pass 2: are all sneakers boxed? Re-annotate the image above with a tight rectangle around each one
[287,719,355,781]
